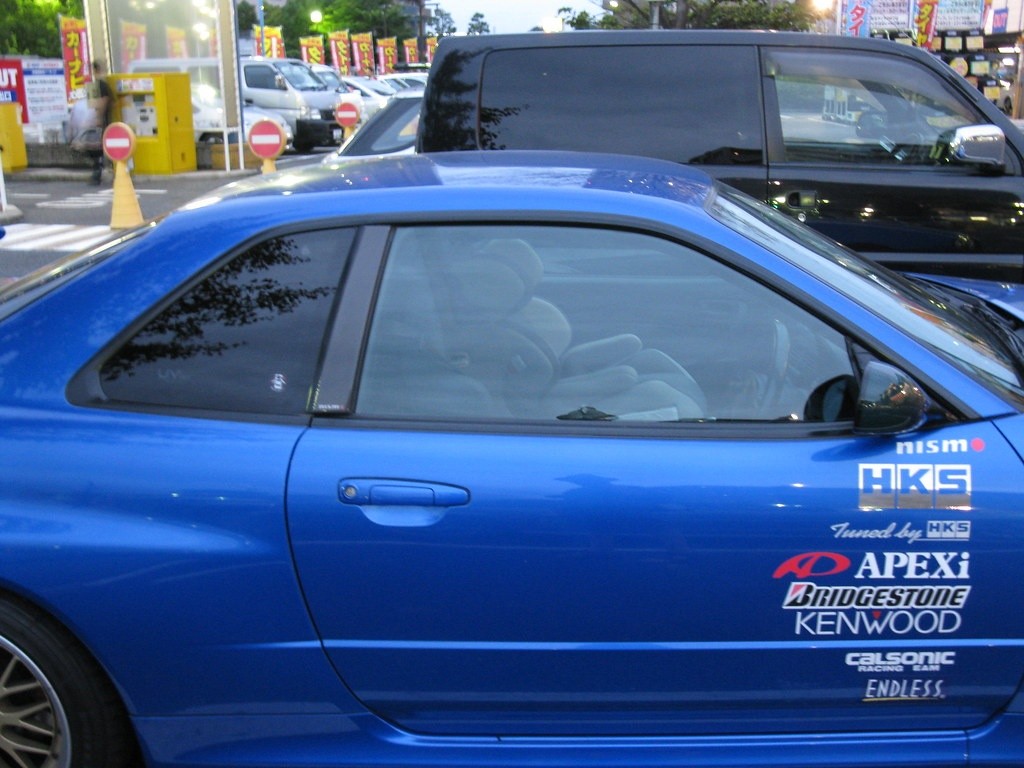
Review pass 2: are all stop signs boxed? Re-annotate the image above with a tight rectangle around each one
[334,103,360,130]
[104,124,134,161]
[248,120,283,160]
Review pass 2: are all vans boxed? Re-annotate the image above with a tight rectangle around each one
[126,57,323,156]
[414,28,1024,284]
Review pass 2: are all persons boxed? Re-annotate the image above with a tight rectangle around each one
[85,60,113,183]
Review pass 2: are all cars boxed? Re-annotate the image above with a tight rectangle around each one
[0,150,1023,768]
[292,72,431,150]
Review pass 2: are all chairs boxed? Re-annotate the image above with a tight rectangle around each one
[442,260,702,424]
[484,234,707,406]
